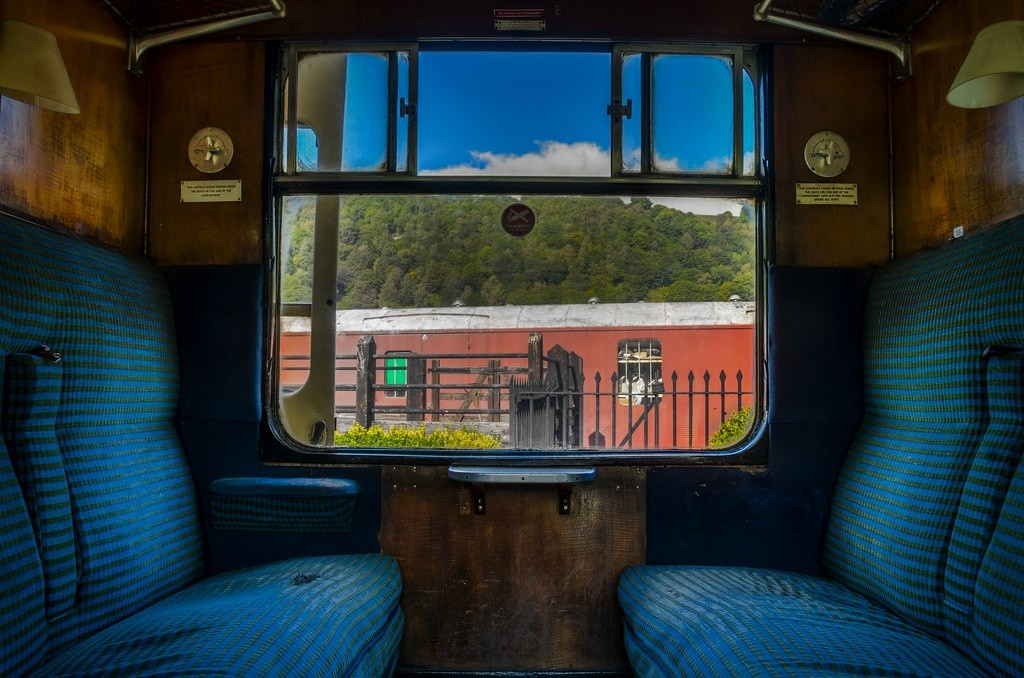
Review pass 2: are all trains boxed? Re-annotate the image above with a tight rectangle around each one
[271,294,766,449]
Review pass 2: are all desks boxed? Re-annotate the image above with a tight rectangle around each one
[448,464,596,514]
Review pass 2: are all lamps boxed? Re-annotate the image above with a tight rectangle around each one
[946,21,1024,110]
[0,19,80,115]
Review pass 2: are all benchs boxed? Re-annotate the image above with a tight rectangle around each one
[0,202,405,678]
[617,215,1024,678]
